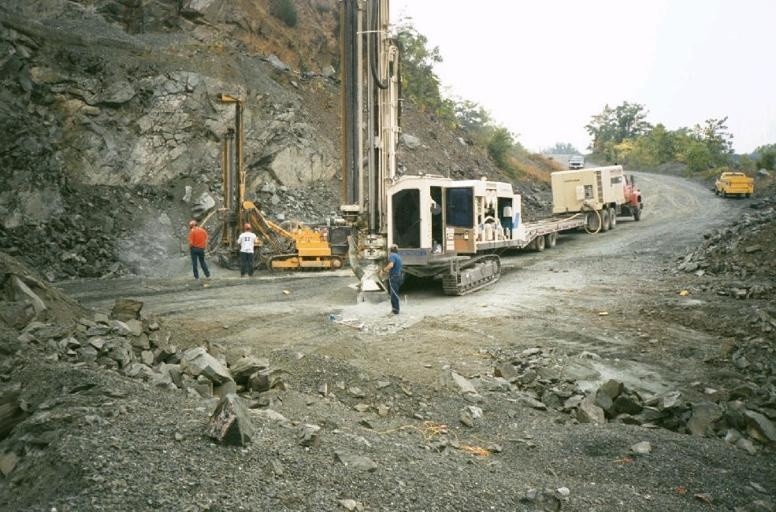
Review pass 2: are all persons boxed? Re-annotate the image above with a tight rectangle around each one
[502,202,513,240]
[383,244,402,315]
[188,221,212,280]
[237,223,257,277]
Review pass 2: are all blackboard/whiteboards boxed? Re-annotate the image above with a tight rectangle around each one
[202,93,348,271]
[551,163,643,232]
[338,1,585,297]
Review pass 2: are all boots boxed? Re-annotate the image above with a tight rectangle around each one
[569,155,584,169]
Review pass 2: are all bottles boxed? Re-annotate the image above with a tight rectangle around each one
[244,224,251,230]
[390,244,399,250]
[189,221,196,225]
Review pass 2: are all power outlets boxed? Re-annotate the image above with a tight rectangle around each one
[715,171,754,198]
